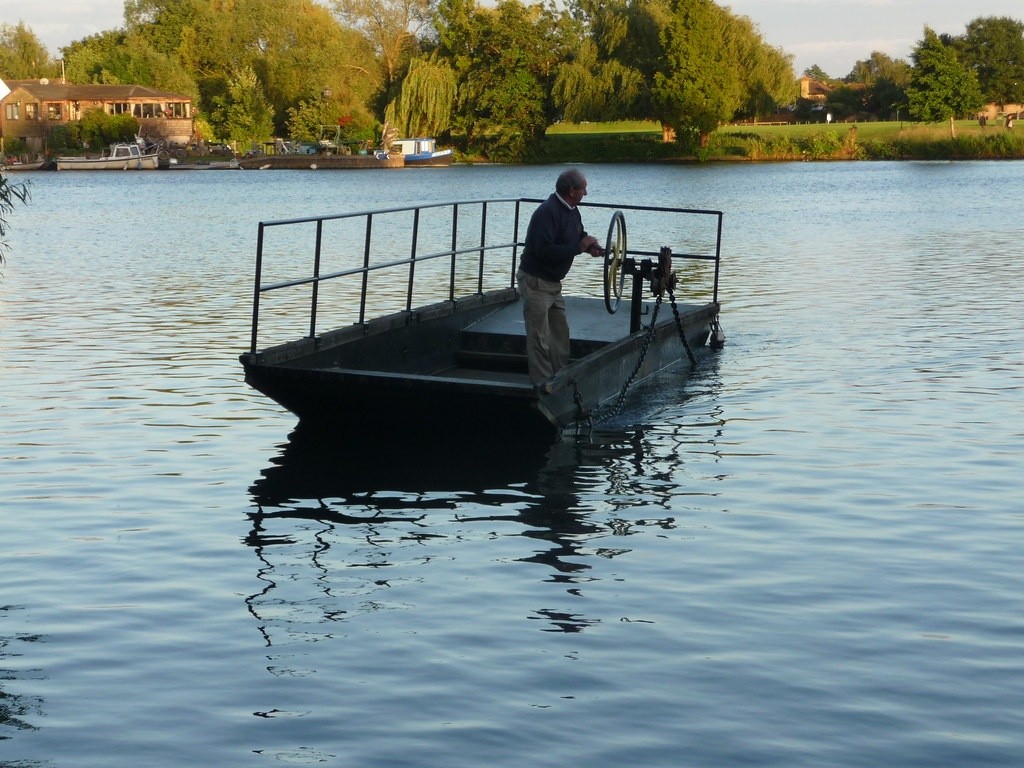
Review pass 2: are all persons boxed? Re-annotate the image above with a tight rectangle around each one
[979,113,987,133]
[1006,115,1012,132]
[516,168,604,388]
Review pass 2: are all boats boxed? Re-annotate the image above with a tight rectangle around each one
[0,154,51,170]
[239,198,723,442]
[237,139,453,168]
[56,144,160,171]
